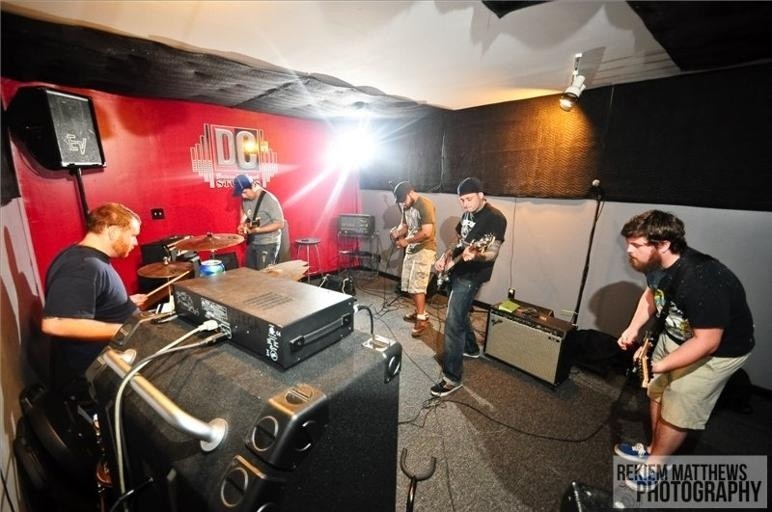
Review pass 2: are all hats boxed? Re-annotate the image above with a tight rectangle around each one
[232,173,254,197]
[457,176,492,197]
[393,180,413,204]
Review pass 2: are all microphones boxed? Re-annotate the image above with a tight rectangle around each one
[591,178,609,198]
[386,177,401,185]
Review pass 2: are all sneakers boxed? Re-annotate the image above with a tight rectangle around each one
[411,319,432,337]
[430,376,463,398]
[403,308,430,323]
[613,440,653,463]
[624,458,674,494]
[462,348,481,359]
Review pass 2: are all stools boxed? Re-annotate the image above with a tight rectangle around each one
[295,239,327,287]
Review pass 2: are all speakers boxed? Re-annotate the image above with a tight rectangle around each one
[6,84,107,170]
[396,271,438,298]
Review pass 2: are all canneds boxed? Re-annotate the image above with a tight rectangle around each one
[199,259,225,277]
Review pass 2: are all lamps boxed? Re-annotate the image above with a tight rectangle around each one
[560,51,587,112]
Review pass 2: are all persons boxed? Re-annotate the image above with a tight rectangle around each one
[389,181,437,337]
[232,174,285,270]
[429,176,507,398]
[614,210,755,493]
[34,202,148,399]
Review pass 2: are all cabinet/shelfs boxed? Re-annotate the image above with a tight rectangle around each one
[336,231,382,283]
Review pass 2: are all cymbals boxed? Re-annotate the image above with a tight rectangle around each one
[261,260,310,281]
[137,262,194,279]
[174,232,245,251]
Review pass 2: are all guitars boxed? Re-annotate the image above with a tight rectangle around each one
[433,233,496,291]
[238,219,261,242]
[624,328,659,390]
